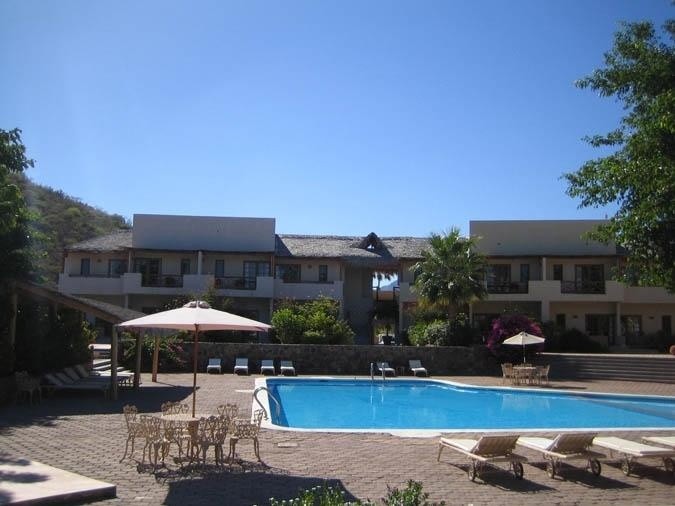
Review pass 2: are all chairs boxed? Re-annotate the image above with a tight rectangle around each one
[14,362,143,409]
[375,359,429,379]
[499,362,551,387]
[437,427,675,485]
[120,398,273,490]
[205,357,297,377]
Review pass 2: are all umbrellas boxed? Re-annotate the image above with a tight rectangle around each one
[114,300,275,418]
[502,331,546,367]
